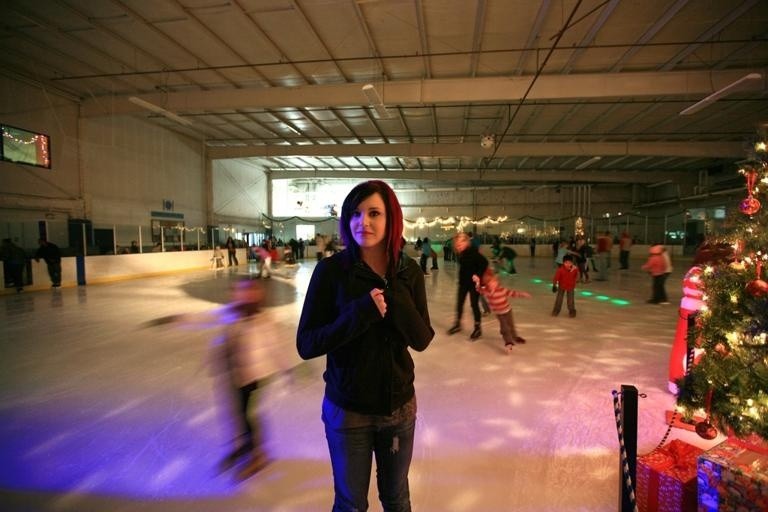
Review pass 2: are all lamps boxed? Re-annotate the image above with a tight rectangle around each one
[676,27,763,119]
[362,47,391,121]
[125,55,195,128]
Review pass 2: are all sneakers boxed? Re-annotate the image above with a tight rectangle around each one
[505,337,526,346]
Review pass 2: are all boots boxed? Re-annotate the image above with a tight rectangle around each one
[470,325,485,338]
[447,320,462,334]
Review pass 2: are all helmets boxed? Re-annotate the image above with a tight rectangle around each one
[648,245,667,254]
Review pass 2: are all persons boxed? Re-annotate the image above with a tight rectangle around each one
[34,238,62,286]
[2,238,25,291]
[296,180,434,512]
[151,243,162,252]
[116,240,139,254]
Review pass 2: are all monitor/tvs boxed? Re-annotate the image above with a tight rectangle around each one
[0,124,51,169]
